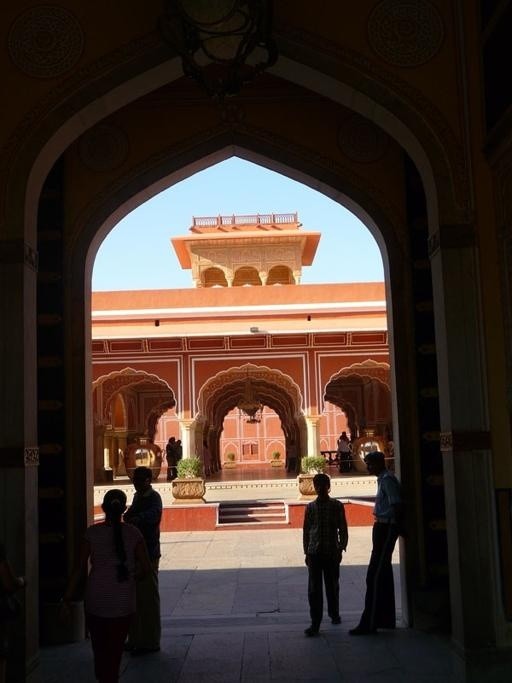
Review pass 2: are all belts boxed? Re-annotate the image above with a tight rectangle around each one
[375,516,398,524]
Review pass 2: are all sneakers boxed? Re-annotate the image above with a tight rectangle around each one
[304,626,321,637]
[331,614,343,624]
[347,628,378,635]
[122,639,162,658]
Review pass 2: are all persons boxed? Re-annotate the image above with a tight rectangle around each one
[165,437,182,480]
[123,466,162,658]
[75,490,149,683]
[203,440,211,476]
[347,450,406,636]
[302,473,348,637]
[336,432,356,473]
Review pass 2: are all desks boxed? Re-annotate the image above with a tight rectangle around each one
[319,450,339,464]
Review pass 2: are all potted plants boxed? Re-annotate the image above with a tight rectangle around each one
[222,452,238,468]
[295,453,332,502]
[271,449,284,467]
[171,456,207,505]
